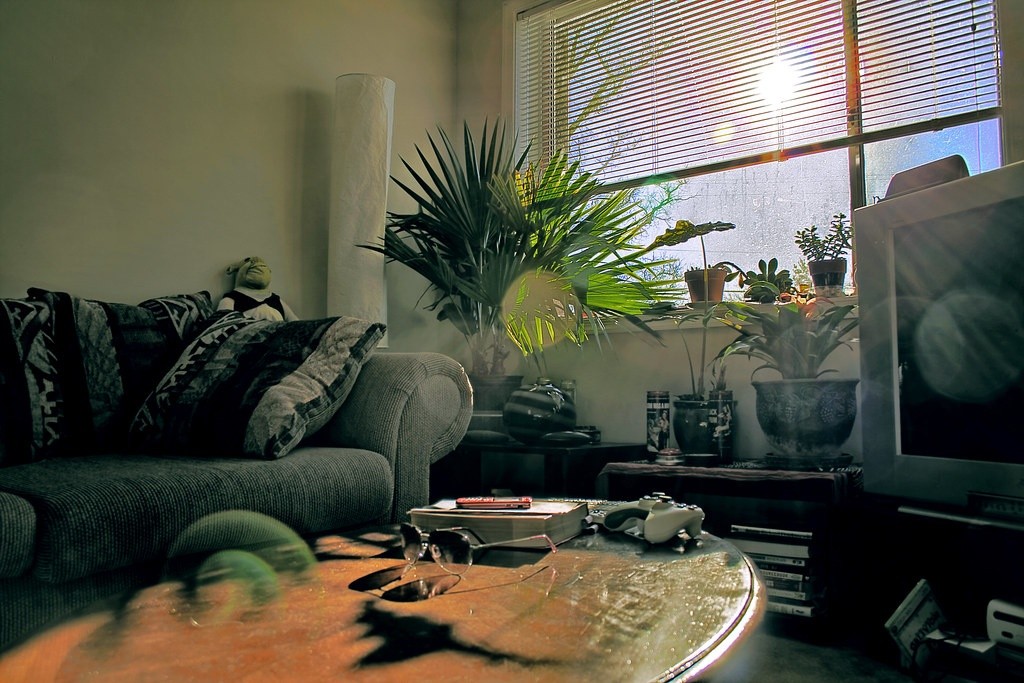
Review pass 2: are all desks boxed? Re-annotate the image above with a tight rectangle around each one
[0,511,769,683]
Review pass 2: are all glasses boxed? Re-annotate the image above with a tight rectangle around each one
[400,524,555,575]
[347,561,549,602]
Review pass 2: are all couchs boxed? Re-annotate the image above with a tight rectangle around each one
[0,299,473,641]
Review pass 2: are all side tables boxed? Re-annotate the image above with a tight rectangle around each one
[450,437,651,498]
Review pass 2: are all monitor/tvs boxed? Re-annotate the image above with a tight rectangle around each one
[850,160,1024,530]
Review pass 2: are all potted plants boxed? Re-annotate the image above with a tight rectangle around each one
[796,213,853,288]
[683,261,748,309]
[711,291,861,470]
[655,218,752,470]
[352,111,692,421]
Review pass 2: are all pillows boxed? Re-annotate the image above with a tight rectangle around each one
[0,259,389,456]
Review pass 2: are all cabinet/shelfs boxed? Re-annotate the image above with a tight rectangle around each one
[861,491,1023,683]
[601,454,865,638]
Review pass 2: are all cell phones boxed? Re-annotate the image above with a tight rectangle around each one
[456,496,532,510]
